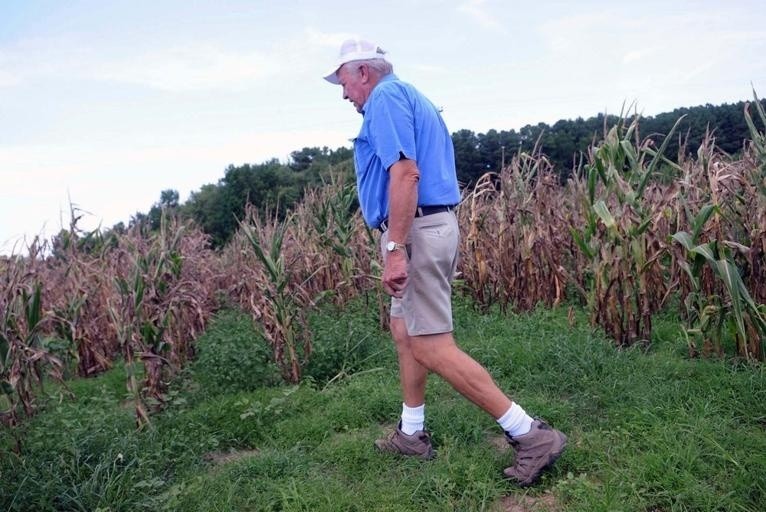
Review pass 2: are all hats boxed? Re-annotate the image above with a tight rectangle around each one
[324,38,389,84]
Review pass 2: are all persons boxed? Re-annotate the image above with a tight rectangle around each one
[323,37,568,488]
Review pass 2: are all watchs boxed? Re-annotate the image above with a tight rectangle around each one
[385,241,406,252]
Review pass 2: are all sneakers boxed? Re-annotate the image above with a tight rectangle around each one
[375,420,432,458]
[503,418,567,486]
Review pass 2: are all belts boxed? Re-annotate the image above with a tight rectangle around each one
[378,205,448,233]
